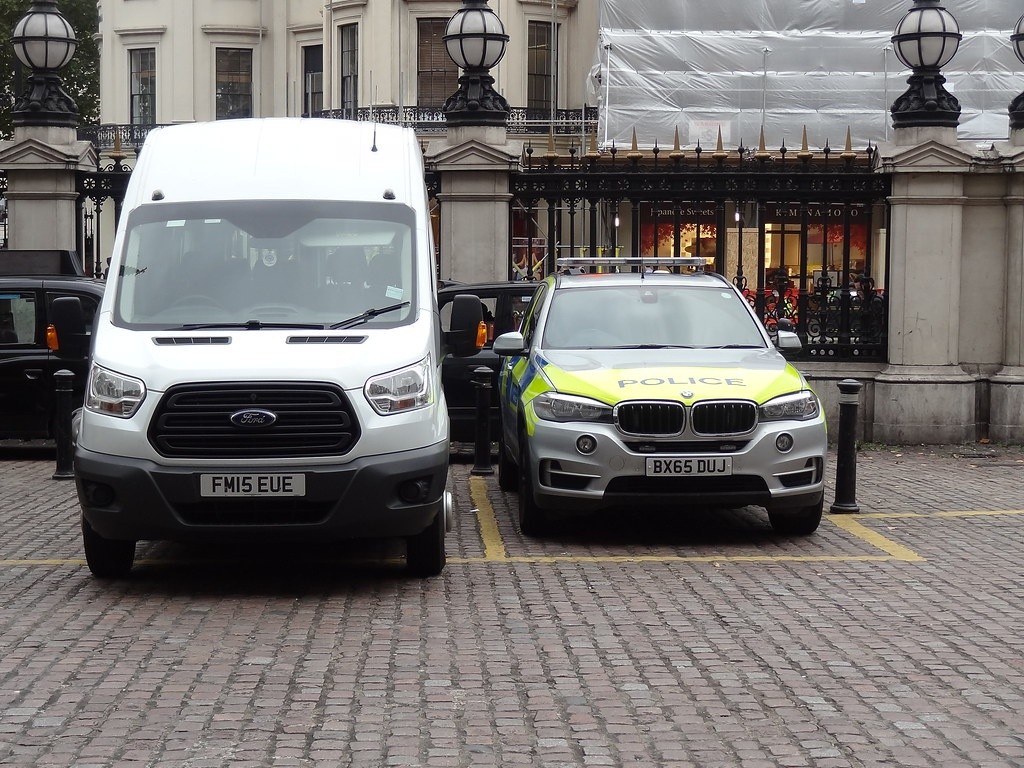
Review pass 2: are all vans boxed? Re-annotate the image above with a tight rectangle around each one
[49,115,493,577]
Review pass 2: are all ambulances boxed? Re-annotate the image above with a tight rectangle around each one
[492,255,831,535]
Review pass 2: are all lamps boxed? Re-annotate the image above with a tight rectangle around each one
[891,0,963,127]
[1007,12,1024,128]
[441,0,514,127]
[9,0,81,127]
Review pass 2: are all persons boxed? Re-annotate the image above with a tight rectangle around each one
[853,276,885,357]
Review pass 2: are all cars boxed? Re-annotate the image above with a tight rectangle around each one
[430,280,543,449]
[0,274,107,444]
